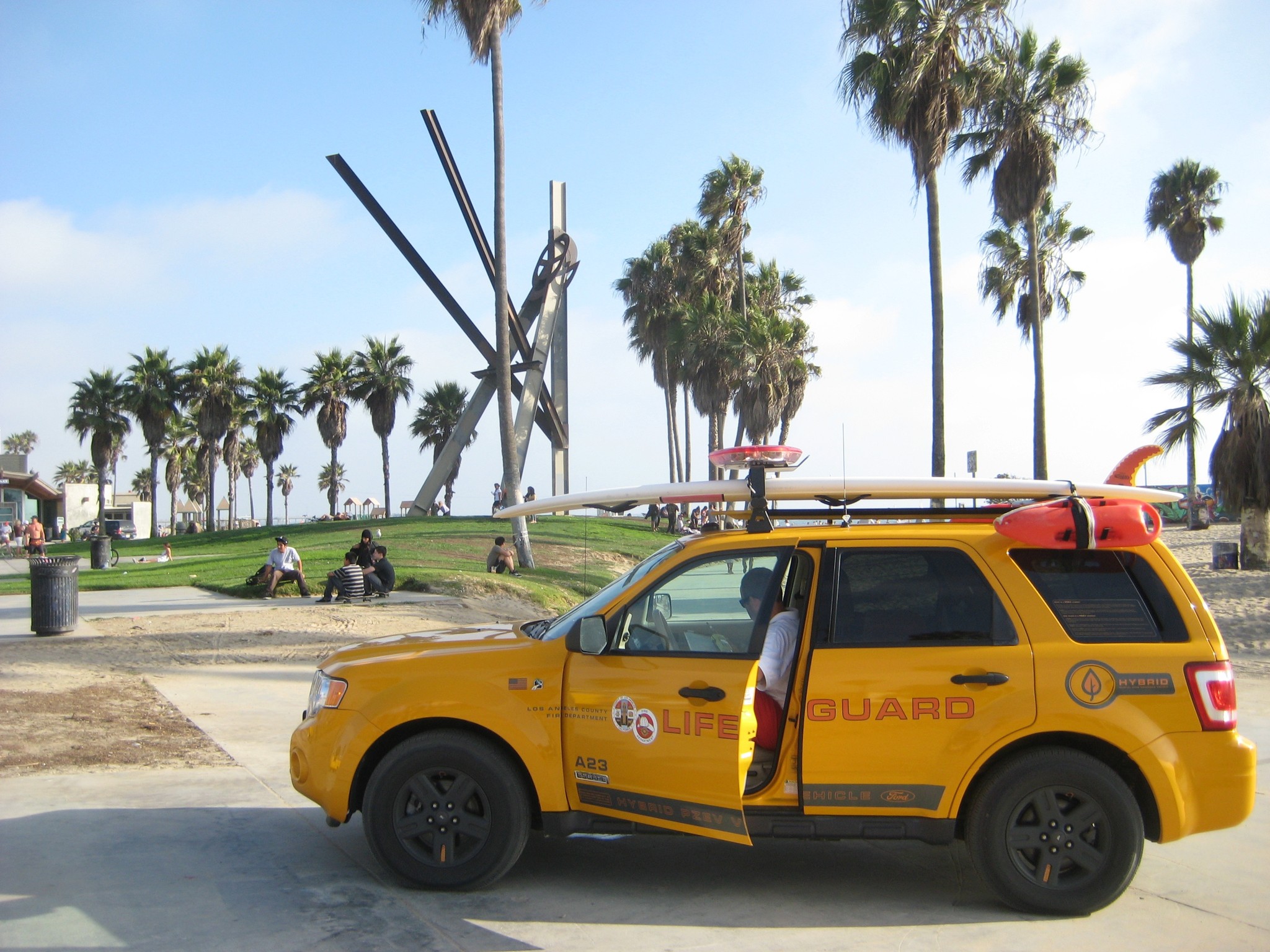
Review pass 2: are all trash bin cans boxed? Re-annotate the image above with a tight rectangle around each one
[88,535,113,569]
[26,555,82,637]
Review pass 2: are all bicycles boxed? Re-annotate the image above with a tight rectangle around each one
[0,536,16,560]
[302,514,321,523]
[106,530,119,566]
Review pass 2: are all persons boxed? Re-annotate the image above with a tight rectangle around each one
[741,557,754,573]
[363,546,395,596]
[349,528,378,565]
[645,500,744,534]
[784,519,910,526]
[257,537,312,599]
[316,512,351,521]
[60,524,67,543]
[487,536,522,578]
[726,559,734,574]
[314,552,365,602]
[708,566,802,751]
[157,525,172,537]
[90,521,100,535]
[432,499,450,516]
[132,542,173,564]
[489,482,538,524]
[0,515,48,563]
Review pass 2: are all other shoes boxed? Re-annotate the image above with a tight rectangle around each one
[133,559,138,564]
[530,521,534,523]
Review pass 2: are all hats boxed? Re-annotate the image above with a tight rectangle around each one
[362,530,370,538]
[4,522,8,526]
[23,520,30,525]
[275,536,288,544]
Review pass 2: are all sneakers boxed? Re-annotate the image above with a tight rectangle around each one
[301,591,311,598]
[316,596,331,602]
[263,593,272,600]
[509,569,522,578]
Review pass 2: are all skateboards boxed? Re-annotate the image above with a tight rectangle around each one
[338,594,377,604]
[260,574,305,585]
[375,592,390,599]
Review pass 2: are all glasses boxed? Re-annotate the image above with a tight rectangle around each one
[739,599,749,608]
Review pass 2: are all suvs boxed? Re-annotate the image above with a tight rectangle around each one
[68,519,100,538]
[288,446,1260,921]
[105,518,137,540]
